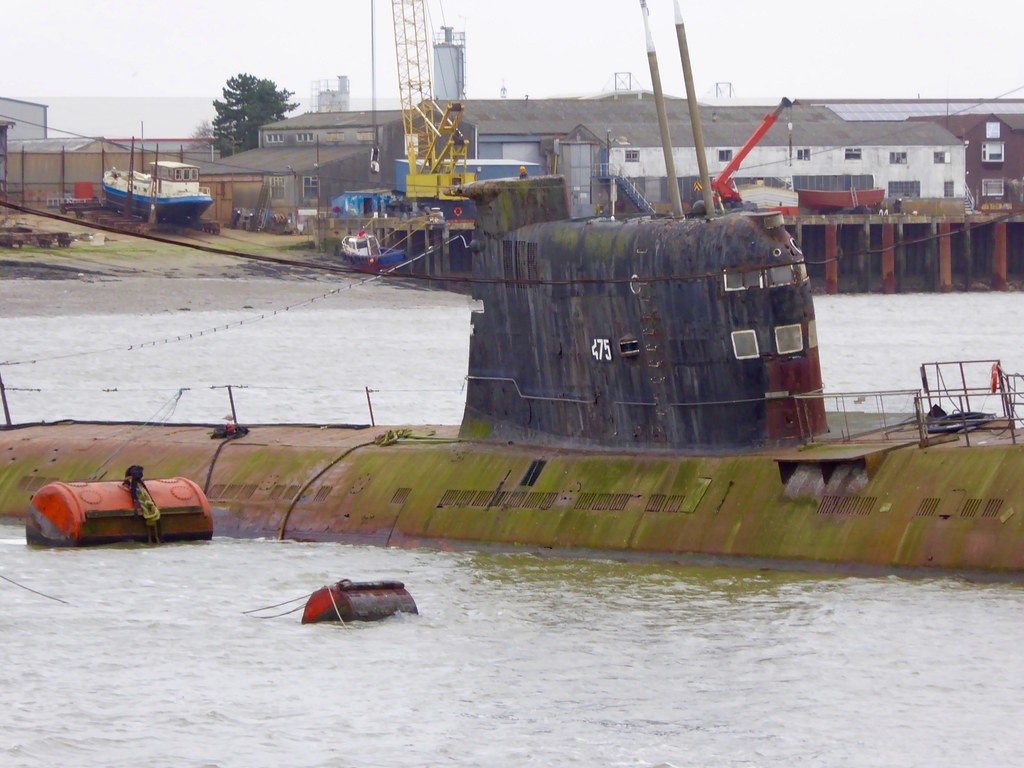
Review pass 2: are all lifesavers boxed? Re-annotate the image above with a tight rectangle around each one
[454,207,462,216]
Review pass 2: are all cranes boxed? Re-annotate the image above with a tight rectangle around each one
[392,0,476,201]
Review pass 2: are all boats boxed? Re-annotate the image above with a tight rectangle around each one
[342,234,404,272]
[738,186,799,217]
[102,161,214,226]
[796,187,886,211]
